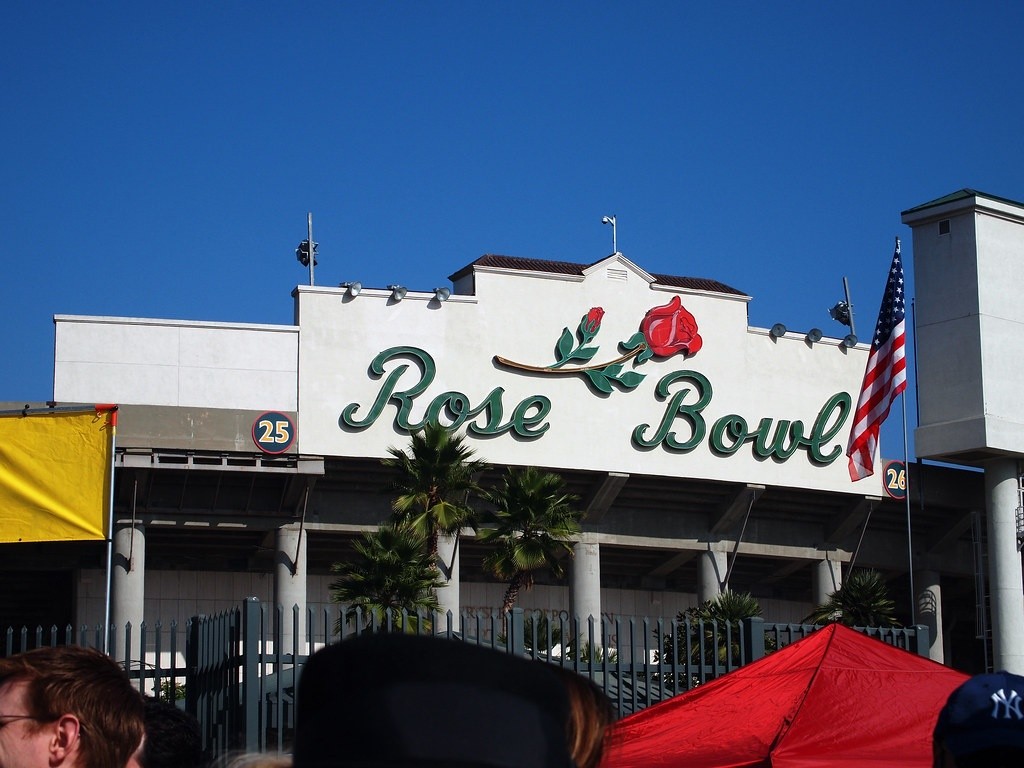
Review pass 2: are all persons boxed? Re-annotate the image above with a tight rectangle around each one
[932,670,1024,768]
[0,632,615,768]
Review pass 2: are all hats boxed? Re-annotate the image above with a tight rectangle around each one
[934,670,1024,768]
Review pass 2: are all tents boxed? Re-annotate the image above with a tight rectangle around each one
[599,623,974,768]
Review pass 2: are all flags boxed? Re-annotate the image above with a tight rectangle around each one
[845,240,906,482]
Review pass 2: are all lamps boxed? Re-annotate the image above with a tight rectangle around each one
[807,327,822,342]
[844,335,858,348]
[433,287,450,302]
[339,281,361,296]
[771,323,786,337]
[387,285,407,301]
